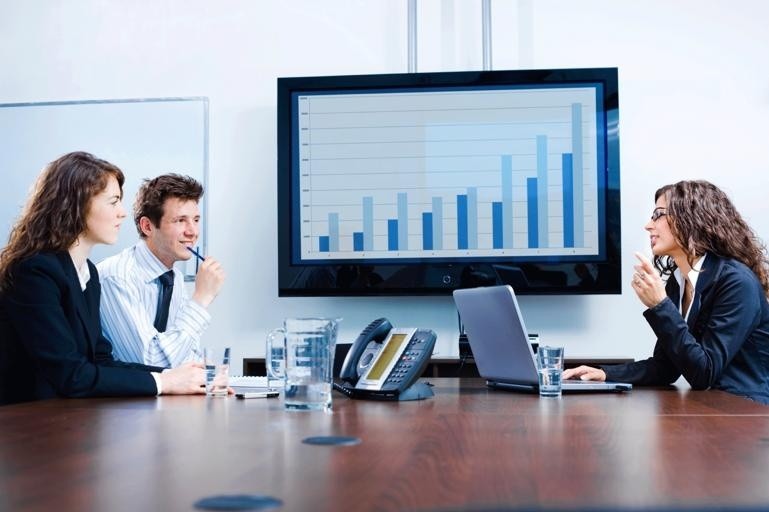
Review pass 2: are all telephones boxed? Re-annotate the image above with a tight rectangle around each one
[339,317,439,401]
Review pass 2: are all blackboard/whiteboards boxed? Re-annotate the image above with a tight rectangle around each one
[0,95,211,280]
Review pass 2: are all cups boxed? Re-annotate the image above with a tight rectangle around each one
[268,317,341,412]
[203,347,230,397]
[539,346,564,398]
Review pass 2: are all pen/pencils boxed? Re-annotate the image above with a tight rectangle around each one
[235,392,280,399]
[187,246,205,261]
[190,349,205,359]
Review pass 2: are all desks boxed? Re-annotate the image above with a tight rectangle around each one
[0,377,766,512]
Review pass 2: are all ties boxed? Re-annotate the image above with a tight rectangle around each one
[152,269,176,334]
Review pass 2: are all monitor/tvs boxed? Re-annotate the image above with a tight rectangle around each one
[277,67,621,298]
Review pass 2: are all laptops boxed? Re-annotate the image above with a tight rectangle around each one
[453,285,633,392]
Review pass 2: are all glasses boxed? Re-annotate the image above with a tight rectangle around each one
[650,210,673,222]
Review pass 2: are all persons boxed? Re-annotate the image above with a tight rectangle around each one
[562,179,769,406]
[92,173,234,395]
[1,151,233,400]
